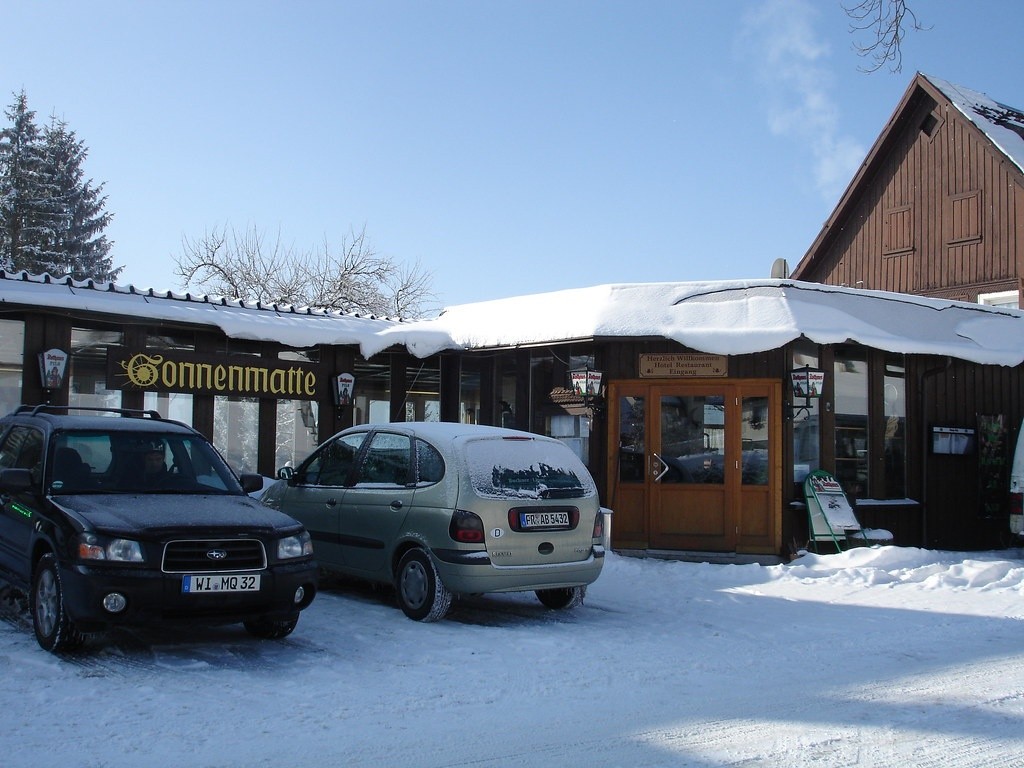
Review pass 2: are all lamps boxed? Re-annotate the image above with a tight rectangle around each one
[35,344,72,404]
[331,369,355,420]
[567,362,608,412]
[783,363,833,422]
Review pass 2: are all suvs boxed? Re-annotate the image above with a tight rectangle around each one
[0,403,321,654]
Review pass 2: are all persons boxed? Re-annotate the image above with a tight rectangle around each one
[117,439,196,495]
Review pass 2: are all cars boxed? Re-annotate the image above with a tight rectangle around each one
[256,423,608,625]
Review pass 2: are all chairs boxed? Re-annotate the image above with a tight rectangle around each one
[55,448,84,488]
